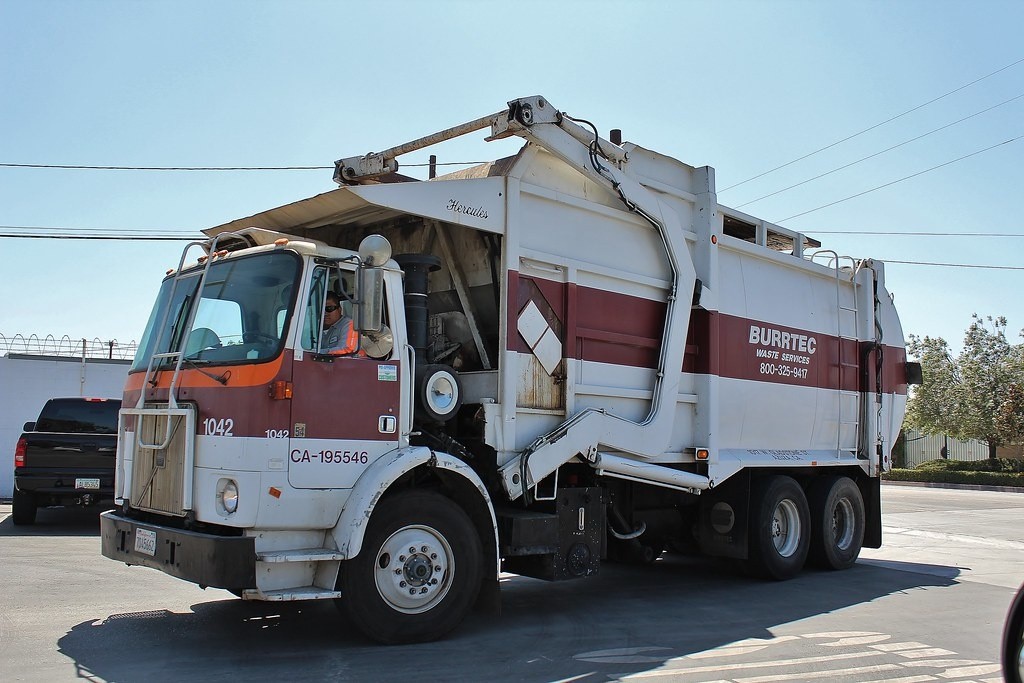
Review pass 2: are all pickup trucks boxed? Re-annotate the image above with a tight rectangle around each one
[11,397,123,527]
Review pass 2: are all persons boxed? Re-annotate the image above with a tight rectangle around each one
[313,289,359,359]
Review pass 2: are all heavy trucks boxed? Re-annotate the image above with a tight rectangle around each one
[95,92,924,646]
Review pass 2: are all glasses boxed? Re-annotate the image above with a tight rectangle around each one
[321,305,339,312]
